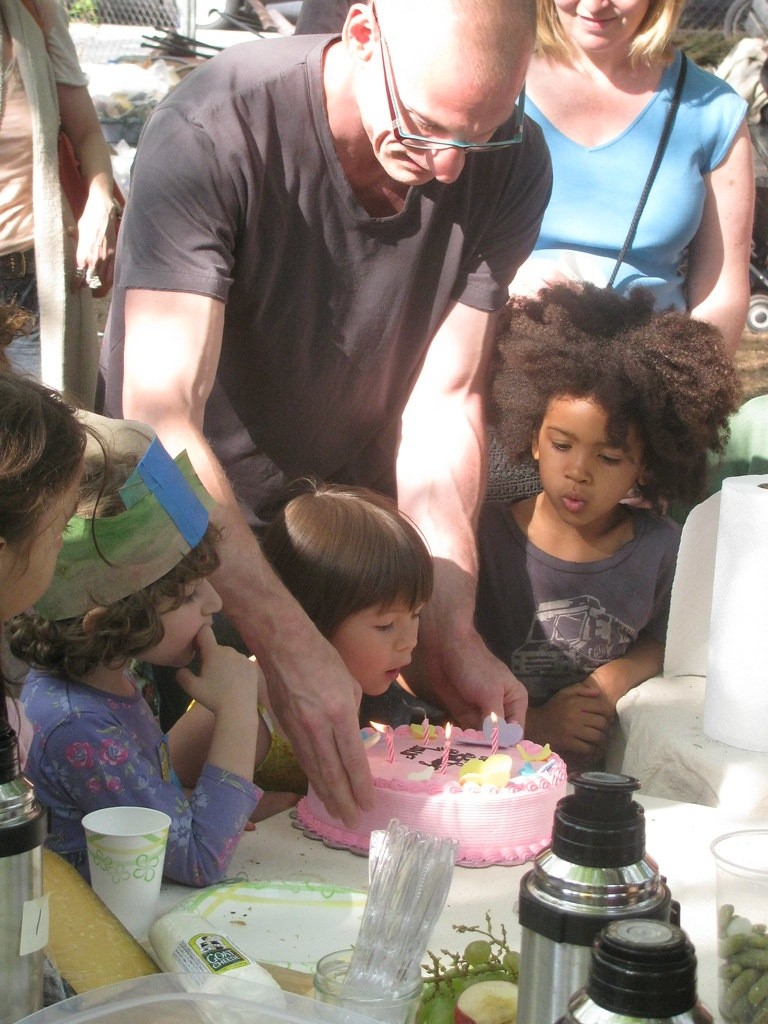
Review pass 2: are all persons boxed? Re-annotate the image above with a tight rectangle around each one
[0,0,755,886]
[94,1,555,830]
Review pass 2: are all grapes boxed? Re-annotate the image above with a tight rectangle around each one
[415,941,523,1024]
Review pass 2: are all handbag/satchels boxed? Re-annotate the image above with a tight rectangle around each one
[482,422,544,504]
[58,129,121,298]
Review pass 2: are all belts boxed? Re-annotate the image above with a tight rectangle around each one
[0,250,36,280]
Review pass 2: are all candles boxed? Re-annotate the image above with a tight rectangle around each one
[439,721,453,779]
[489,709,500,756]
[368,719,394,765]
[422,713,430,747]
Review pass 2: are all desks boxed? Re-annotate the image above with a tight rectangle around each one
[603,673,768,815]
[163,780,768,1024]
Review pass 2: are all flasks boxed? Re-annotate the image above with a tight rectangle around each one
[1,724,47,1023]
[517,768,680,1024]
[553,917,717,1024]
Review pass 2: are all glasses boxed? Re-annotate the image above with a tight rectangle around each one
[371,2,526,152]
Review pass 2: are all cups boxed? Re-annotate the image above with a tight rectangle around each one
[311,947,424,1023]
[82,805,172,941]
[710,829,768,1024]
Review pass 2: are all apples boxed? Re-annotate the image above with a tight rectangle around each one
[454,980,519,1024]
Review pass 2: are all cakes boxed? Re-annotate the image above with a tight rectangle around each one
[294,724,568,863]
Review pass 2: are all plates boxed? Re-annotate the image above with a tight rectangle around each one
[168,880,368,972]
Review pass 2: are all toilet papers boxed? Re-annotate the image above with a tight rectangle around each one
[663,473,767,754]
